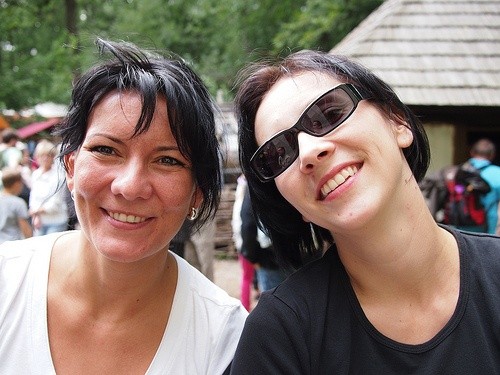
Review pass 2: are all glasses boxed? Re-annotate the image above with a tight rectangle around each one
[247,81,372,185]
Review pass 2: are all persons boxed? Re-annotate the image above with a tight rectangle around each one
[277,146,291,169]
[230,173,283,315]
[312,120,323,134]
[222,49,500,375]
[0,39,251,375]
[0,129,78,243]
[452,139,500,236]
[173,207,216,282]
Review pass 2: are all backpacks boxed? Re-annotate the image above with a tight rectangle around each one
[452,161,494,234]
